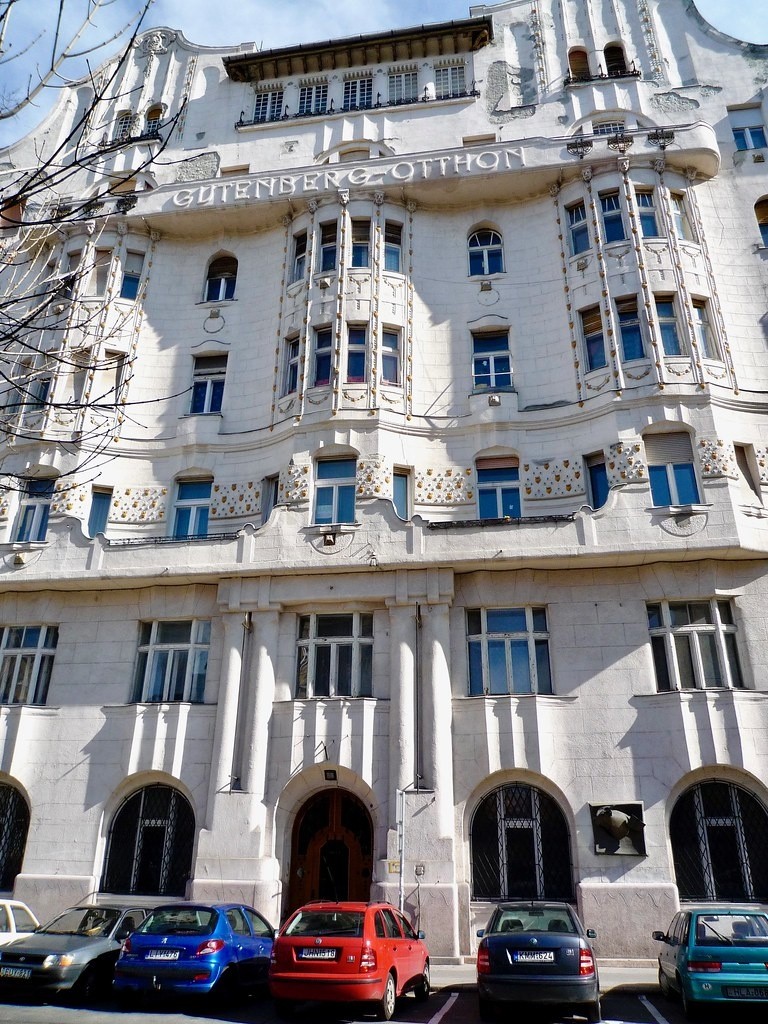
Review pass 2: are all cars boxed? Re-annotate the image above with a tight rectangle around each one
[652,907,768,1022]
[0,898,42,947]
[0,903,171,1009]
[112,899,280,1010]
[476,900,601,1024]
[267,900,430,1021]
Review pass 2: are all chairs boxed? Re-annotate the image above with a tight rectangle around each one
[732,922,750,935]
[120,915,135,934]
[698,923,705,938]
[501,920,525,932]
[546,920,568,932]
